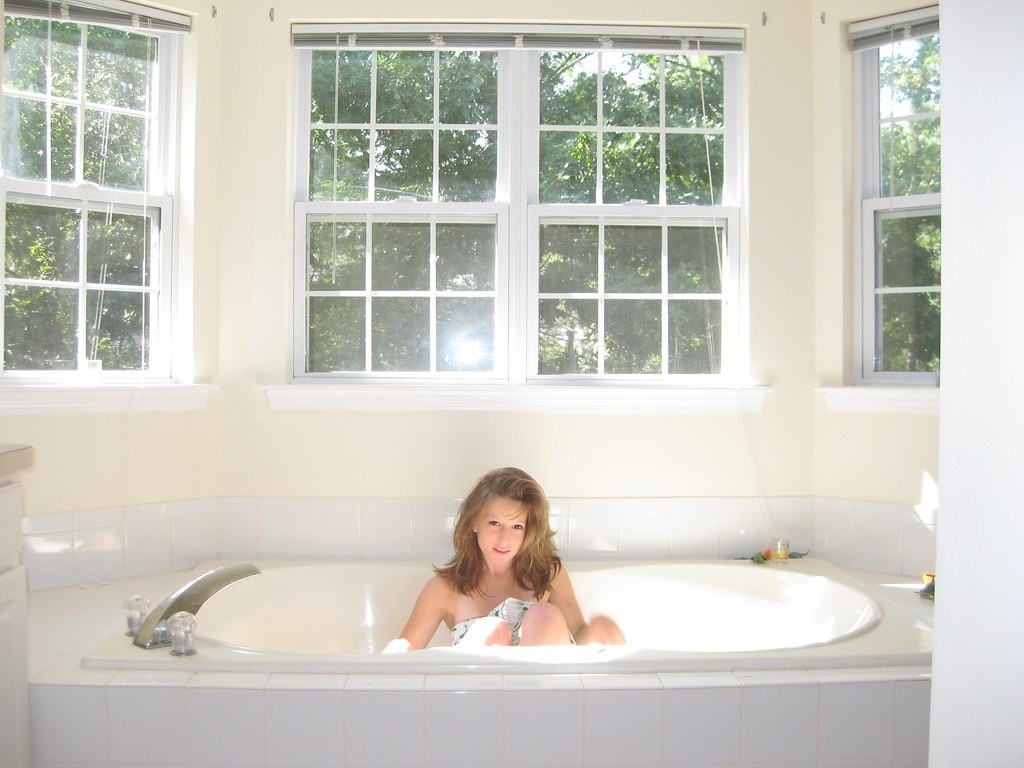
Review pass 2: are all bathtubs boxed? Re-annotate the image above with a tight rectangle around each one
[28,557,933,768]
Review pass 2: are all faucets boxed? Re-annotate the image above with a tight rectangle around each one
[133,561,261,650]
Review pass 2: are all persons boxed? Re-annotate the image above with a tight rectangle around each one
[399,468,626,654]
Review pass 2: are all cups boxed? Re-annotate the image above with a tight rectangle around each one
[771,537,790,562]
